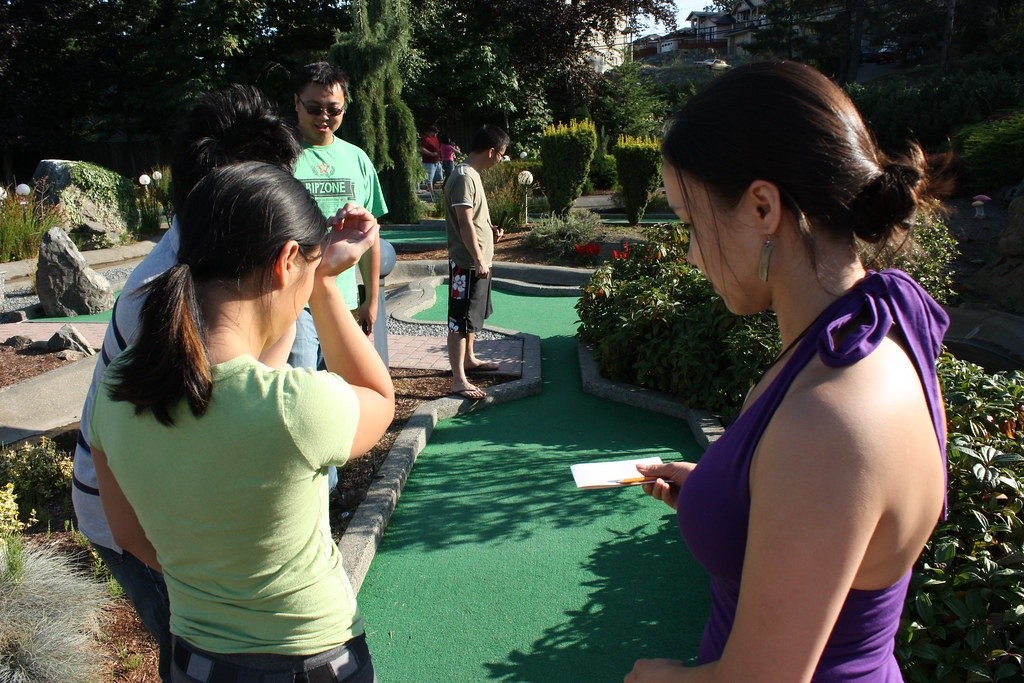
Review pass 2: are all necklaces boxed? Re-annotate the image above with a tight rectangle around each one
[743,310,826,406]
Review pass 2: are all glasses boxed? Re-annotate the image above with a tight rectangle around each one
[297,94,346,116]
[492,147,506,161]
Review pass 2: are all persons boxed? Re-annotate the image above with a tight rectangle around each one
[625,60,951,683]
[71,62,395,683]
[418,123,509,399]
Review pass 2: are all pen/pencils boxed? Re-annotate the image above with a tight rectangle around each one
[616,476,659,484]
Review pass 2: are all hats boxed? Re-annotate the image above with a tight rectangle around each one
[429,126,439,132]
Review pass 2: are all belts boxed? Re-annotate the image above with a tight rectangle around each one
[173,642,368,683]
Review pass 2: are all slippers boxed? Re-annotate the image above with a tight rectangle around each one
[448,383,486,399]
[464,360,499,371]
